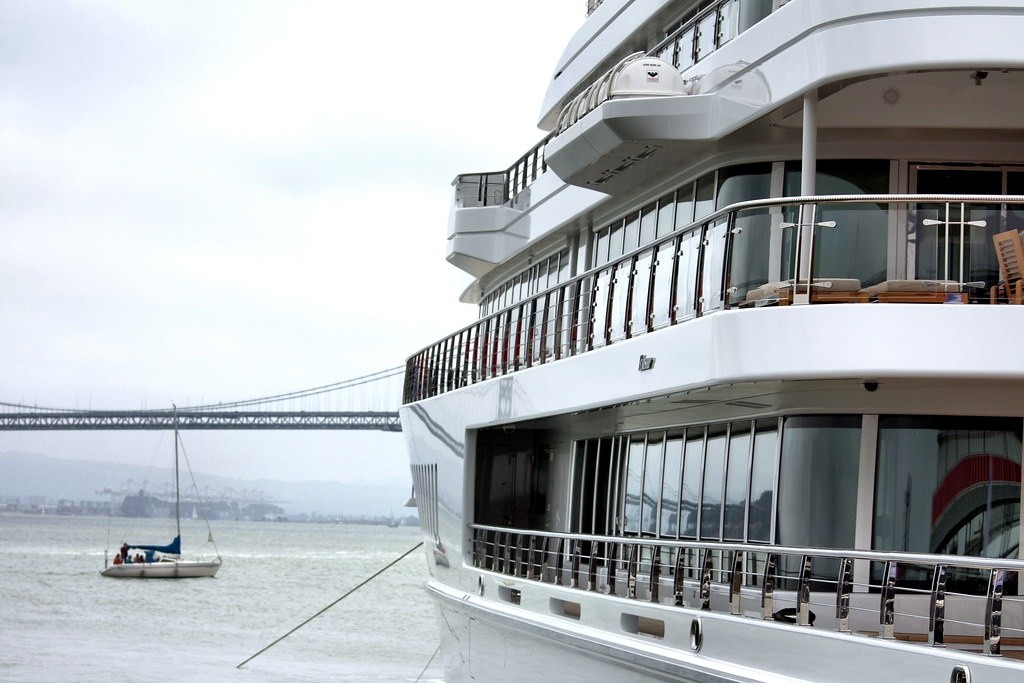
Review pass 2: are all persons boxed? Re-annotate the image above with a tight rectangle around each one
[113,543,144,564]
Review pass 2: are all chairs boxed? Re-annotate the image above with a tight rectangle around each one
[991,229,1024,304]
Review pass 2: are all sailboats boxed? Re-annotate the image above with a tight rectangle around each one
[98,403,224,578]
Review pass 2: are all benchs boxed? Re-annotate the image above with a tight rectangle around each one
[861,279,968,304]
[745,278,869,305]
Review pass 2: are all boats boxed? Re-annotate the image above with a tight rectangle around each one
[399,0,1024,683]
